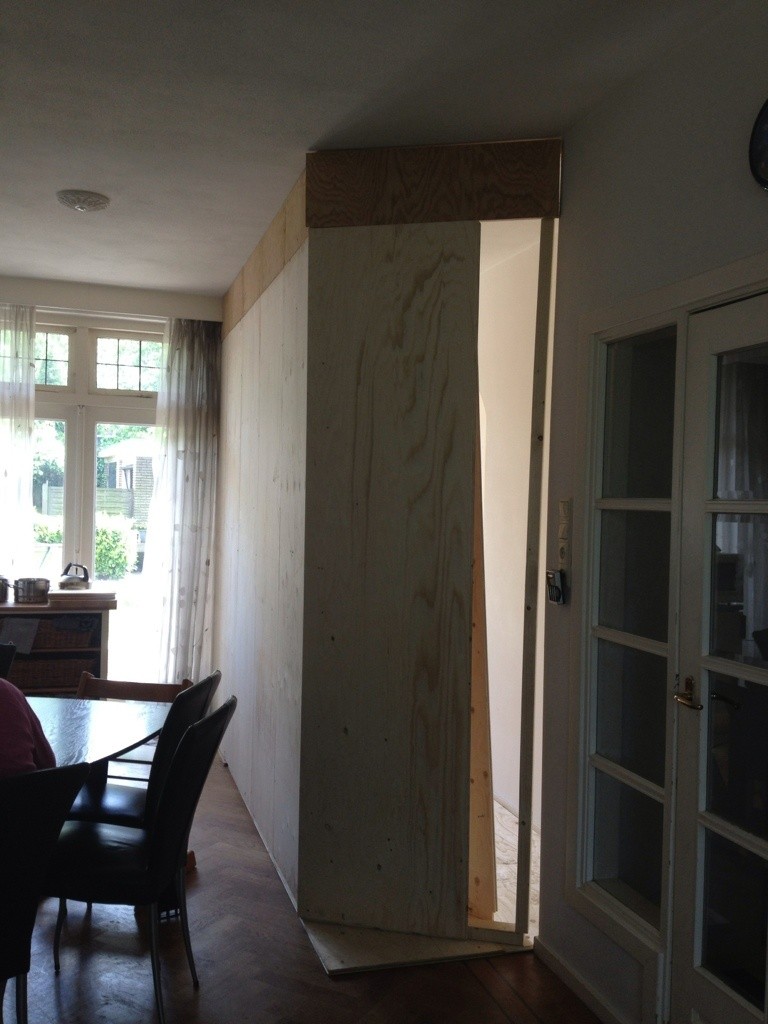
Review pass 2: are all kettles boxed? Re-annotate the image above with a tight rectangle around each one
[59,563,90,590]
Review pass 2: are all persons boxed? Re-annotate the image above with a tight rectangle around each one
[1,677,60,778]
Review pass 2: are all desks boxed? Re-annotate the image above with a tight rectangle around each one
[23,696,171,782]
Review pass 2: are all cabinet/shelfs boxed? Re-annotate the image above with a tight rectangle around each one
[0,602,117,702]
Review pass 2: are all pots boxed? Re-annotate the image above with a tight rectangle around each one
[13,577,50,603]
[0,577,12,603]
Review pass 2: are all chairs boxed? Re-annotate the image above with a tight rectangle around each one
[0,668,238,1024]
